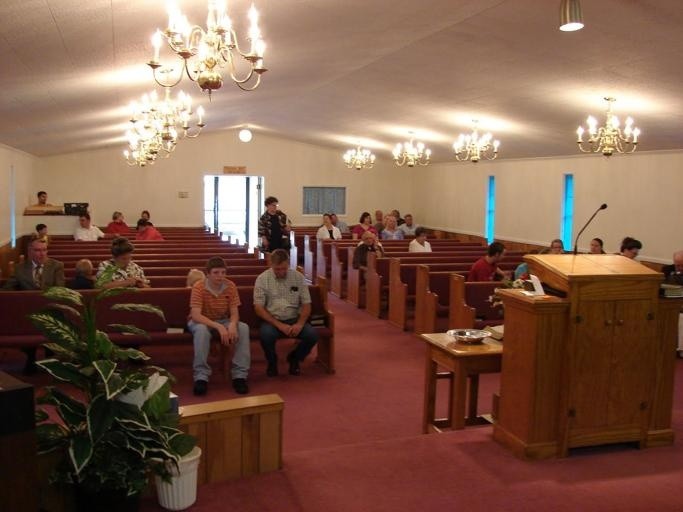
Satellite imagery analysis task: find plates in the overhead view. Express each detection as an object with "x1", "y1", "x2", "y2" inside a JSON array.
[{"x1": 447, "y1": 328, "x2": 492, "y2": 342}]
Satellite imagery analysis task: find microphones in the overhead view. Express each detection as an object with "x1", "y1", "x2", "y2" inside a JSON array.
[{"x1": 572, "y1": 201, "x2": 608, "y2": 253}]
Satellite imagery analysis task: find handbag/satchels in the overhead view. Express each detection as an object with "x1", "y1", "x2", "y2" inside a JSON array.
[{"x1": 279, "y1": 238, "x2": 288, "y2": 247}]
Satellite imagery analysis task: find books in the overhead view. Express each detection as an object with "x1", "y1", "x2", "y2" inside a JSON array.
[
  {"x1": 308, "y1": 313, "x2": 326, "y2": 325},
  {"x1": 661, "y1": 283, "x2": 683, "y2": 296}
]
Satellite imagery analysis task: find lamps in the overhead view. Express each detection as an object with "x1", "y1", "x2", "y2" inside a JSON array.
[
  {"x1": 392, "y1": 130, "x2": 432, "y2": 167},
  {"x1": 576, "y1": 97, "x2": 641, "y2": 158},
  {"x1": 128, "y1": 141, "x2": 177, "y2": 160},
  {"x1": 342, "y1": 141, "x2": 376, "y2": 171},
  {"x1": 238, "y1": 128, "x2": 252, "y2": 143},
  {"x1": 558, "y1": 0, "x2": 585, "y2": 32},
  {"x1": 129, "y1": 87, "x2": 208, "y2": 142},
  {"x1": 122, "y1": 151, "x2": 155, "y2": 168},
  {"x1": 453, "y1": 112, "x2": 501, "y2": 162},
  {"x1": 147, "y1": 1, "x2": 268, "y2": 103}
]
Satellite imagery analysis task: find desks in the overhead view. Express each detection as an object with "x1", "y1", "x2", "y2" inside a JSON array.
[{"x1": 417, "y1": 332, "x2": 504, "y2": 434}]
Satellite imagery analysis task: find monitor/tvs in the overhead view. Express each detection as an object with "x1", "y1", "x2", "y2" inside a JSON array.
[{"x1": 63, "y1": 202, "x2": 89, "y2": 215}]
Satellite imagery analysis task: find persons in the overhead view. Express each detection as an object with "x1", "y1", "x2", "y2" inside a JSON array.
[
  {"x1": 589, "y1": 238, "x2": 606, "y2": 254},
  {"x1": 662, "y1": 250, "x2": 682, "y2": 281},
  {"x1": 186, "y1": 255, "x2": 251, "y2": 396},
  {"x1": 515, "y1": 238, "x2": 565, "y2": 280},
  {"x1": 186, "y1": 268, "x2": 206, "y2": 288},
  {"x1": 464, "y1": 242, "x2": 512, "y2": 319},
  {"x1": 258, "y1": 196, "x2": 292, "y2": 253},
  {"x1": 620, "y1": 236, "x2": 643, "y2": 260},
  {"x1": 0, "y1": 190, "x2": 165, "y2": 378},
  {"x1": 253, "y1": 249, "x2": 319, "y2": 378},
  {"x1": 316, "y1": 209, "x2": 432, "y2": 283}
]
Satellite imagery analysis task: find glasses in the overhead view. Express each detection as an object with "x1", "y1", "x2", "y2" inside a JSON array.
[
  {"x1": 31, "y1": 248, "x2": 47, "y2": 253},
  {"x1": 627, "y1": 249, "x2": 638, "y2": 255}
]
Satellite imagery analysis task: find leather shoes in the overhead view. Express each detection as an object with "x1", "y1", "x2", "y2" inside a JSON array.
[
  {"x1": 266, "y1": 354, "x2": 278, "y2": 377},
  {"x1": 286, "y1": 352, "x2": 300, "y2": 375}
]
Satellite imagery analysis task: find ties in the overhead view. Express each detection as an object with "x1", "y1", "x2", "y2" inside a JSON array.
[
  {"x1": 34, "y1": 266, "x2": 41, "y2": 289},
  {"x1": 269, "y1": 203, "x2": 279, "y2": 207}
]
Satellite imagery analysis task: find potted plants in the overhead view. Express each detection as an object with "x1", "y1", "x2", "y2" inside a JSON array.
[{"x1": 33, "y1": 285, "x2": 202, "y2": 510}]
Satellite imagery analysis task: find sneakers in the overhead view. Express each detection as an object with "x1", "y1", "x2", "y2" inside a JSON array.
[
  {"x1": 193, "y1": 379, "x2": 207, "y2": 395},
  {"x1": 232, "y1": 377, "x2": 248, "y2": 394}
]
